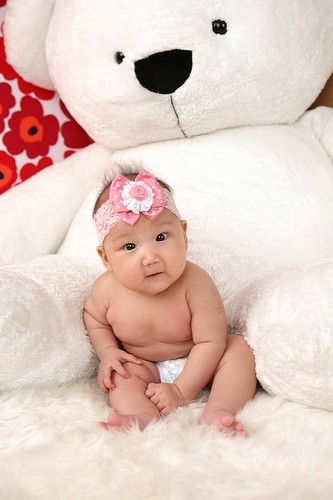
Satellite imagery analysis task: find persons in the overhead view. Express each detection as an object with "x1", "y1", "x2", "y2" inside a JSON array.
[{"x1": 82, "y1": 171, "x2": 257, "y2": 439}]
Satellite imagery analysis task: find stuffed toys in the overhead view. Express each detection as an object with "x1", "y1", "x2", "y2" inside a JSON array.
[{"x1": 0, "y1": 0, "x2": 333, "y2": 414}]
